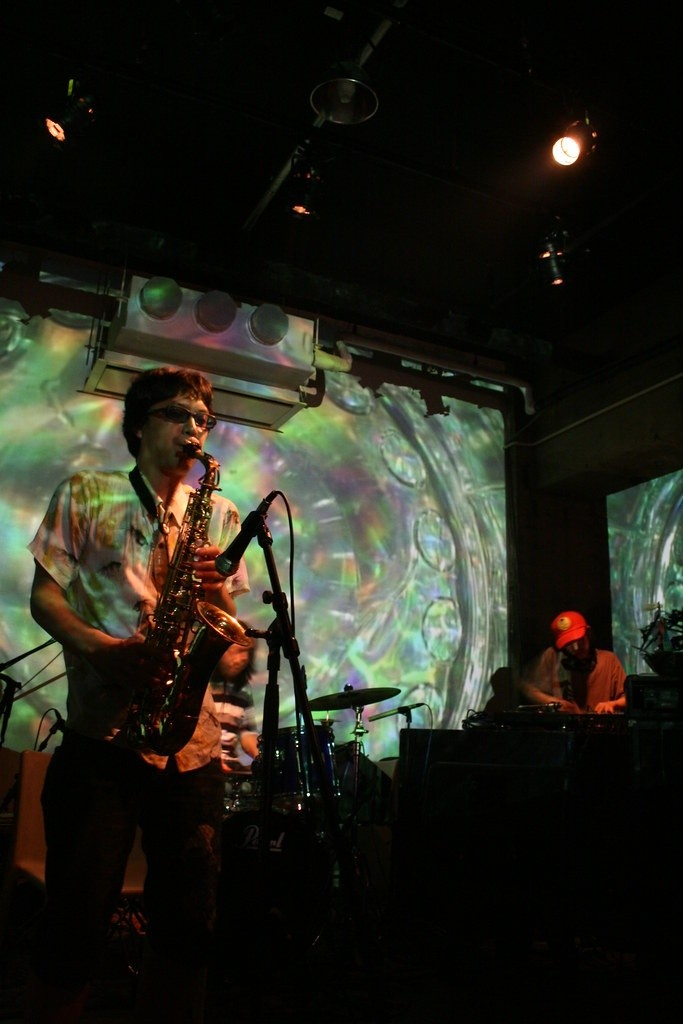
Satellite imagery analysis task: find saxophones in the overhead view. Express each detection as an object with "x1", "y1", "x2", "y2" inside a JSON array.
[{"x1": 115, "y1": 439, "x2": 256, "y2": 755}]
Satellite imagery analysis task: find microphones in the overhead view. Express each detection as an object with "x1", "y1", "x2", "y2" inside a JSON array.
[
  {"x1": 367, "y1": 703, "x2": 424, "y2": 722},
  {"x1": 54, "y1": 709, "x2": 65, "y2": 732},
  {"x1": 215, "y1": 489, "x2": 277, "y2": 577}
]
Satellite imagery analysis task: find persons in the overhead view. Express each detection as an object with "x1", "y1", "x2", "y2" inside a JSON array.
[
  {"x1": 522, "y1": 612, "x2": 626, "y2": 714},
  {"x1": 29, "y1": 367, "x2": 248, "y2": 1023},
  {"x1": 209, "y1": 618, "x2": 263, "y2": 802}
]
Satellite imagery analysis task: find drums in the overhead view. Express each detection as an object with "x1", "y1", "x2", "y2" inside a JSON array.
[
  {"x1": 258, "y1": 725, "x2": 342, "y2": 816},
  {"x1": 221, "y1": 771, "x2": 259, "y2": 813}
]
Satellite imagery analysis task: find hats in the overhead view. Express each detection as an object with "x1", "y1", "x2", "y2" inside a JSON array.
[{"x1": 551, "y1": 611, "x2": 590, "y2": 650}]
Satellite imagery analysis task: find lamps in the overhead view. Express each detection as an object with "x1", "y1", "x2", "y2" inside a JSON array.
[
  {"x1": 531, "y1": 217, "x2": 571, "y2": 286},
  {"x1": 310, "y1": 58, "x2": 380, "y2": 125},
  {"x1": 45, "y1": 70, "x2": 98, "y2": 143},
  {"x1": 289, "y1": 149, "x2": 327, "y2": 216}
]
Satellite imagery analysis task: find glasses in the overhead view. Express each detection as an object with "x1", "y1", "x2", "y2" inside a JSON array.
[{"x1": 147, "y1": 404, "x2": 217, "y2": 429}]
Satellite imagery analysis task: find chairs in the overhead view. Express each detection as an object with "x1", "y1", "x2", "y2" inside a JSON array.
[{"x1": 0, "y1": 751, "x2": 148, "y2": 975}]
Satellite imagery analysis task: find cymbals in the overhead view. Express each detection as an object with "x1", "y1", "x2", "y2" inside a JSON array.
[
  {"x1": 296, "y1": 687, "x2": 401, "y2": 711},
  {"x1": 313, "y1": 718, "x2": 341, "y2": 722}
]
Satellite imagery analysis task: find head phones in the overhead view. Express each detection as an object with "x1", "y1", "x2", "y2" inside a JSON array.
[{"x1": 562, "y1": 647, "x2": 596, "y2": 676}]
